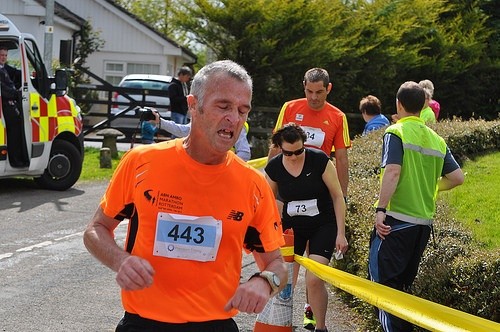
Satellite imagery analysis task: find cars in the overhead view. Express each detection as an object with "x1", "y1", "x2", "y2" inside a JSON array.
[{"x1": 111, "y1": 74, "x2": 191, "y2": 138}]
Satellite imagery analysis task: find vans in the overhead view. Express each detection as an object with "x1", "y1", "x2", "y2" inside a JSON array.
[{"x1": 0, "y1": 12, "x2": 85, "y2": 191}]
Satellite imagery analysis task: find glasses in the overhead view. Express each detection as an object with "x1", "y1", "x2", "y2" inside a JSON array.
[{"x1": 281, "y1": 146, "x2": 304, "y2": 156}]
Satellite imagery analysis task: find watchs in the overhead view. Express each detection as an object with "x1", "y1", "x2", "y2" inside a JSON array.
[
  {"x1": 344, "y1": 196, "x2": 348, "y2": 203},
  {"x1": 249, "y1": 270, "x2": 281, "y2": 296},
  {"x1": 374, "y1": 206, "x2": 386, "y2": 213}
]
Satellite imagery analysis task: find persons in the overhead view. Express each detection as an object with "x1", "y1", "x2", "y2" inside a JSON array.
[
  {"x1": 0, "y1": 48, "x2": 29, "y2": 168},
  {"x1": 418, "y1": 79, "x2": 440, "y2": 120},
  {"x1": 149, "y1": 109, "x2": 252, "y2": 161},
  {"x1": 263, "y1": 124, "x2": 349, "y2": 332},
  {"x1": 167, "y1": 66, "x2": 193, "y2": 139},
  {"x1": 391, "y1": 86, "x2": 436, "y2": 124},
  {"x1": 265, "y1": 67, "x2": 352, "y2": 211},
  {"x1": 367, "y1": 81, "x2": 465, "y2": 332},
  {"x1": 359, "y1": 94, "x2": 391, "y2": 137},
  {"x1": 82, "y1": 59, "x2": 290, "y2": 331}
]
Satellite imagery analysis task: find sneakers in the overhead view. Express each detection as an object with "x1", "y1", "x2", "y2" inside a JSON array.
[{"x1": 303, "y1": 304, "x2": 316, "y2": 330}]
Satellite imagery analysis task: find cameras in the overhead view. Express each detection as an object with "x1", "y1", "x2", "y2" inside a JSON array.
[{"x1": 134, "y1": 106, "x2": 159, "y2": 121}]
